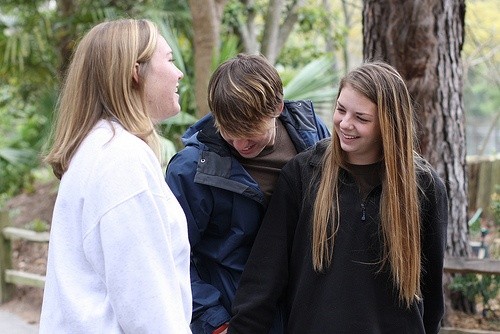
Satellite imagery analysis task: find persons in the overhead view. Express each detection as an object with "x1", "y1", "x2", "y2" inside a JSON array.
[
  {"x1": 37, "y1": 17, "x2": 193, "y2": 333},
  {"x1": 165, "y1": 53, "x2": 331, "y2": 333},
  {"x1": 226, "y1": 58, "x2": 448, "y2": 334}
]
